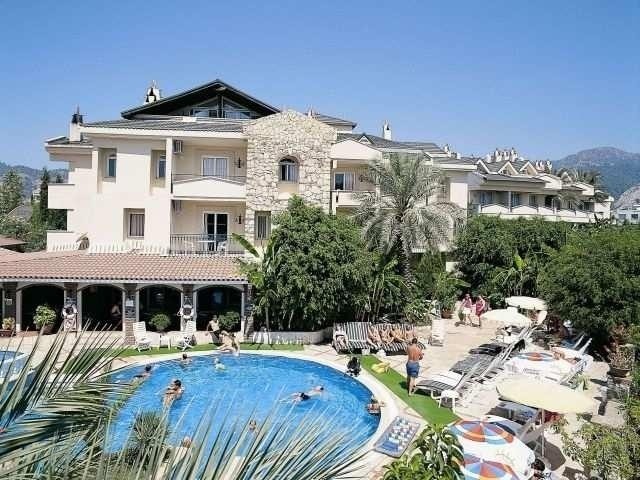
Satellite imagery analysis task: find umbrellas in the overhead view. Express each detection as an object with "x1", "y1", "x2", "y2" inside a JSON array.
[
  {"x1": 479, "y1": 308, "x2": 532, "y2": 352},
  {"x1": 497, "y1": 374, "x2": 602, "y2": 458},
  {"x1": 449, "y1": 417, "x2": 514, "y2": 446},
  {"x1": 505, "y1": 296, "x2": 549, "y2": 317},
  {"x1": 461, "y1": 454, "x2": 516, "y2": 479}
]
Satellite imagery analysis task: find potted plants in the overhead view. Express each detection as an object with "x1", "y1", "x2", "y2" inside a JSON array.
[
  {"x1": 608, "y1": 340, "x2": 636, "y2": 379},
  {"x1": 33, "y1": 305, "x2": 56, "y2": 335},
  {"x1": 0, "y1": 317, "x2": 17, "y2": 337}
]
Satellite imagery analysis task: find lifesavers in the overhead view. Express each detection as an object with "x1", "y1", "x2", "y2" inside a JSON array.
[
  {"x1": 372, "y1": 362, "x2": 390, "y2": 374},
  {"x1": 62, "y1": 304, "x2": 77, "y2": 319},
  {"x1": 136, "y1": 336, "x2": 152, "y2": 344},
  {"x1": 180, "y1": 304, "x2": 194, "y2": 319}
]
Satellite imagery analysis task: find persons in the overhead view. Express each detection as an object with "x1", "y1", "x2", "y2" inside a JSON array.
[
  {"x1": 332, "y1": 322, "x2": 425, "y2": 397},
  {"x1": 204, "y1": 315, "x2": 240, "y2": 357},
  {"x1": 157, "y1": 379, "x2": 183, "y2": 409},
  {"x1": 110, "y1": 303, "x2": 122, "y2": 318},
  {"x1": 181, "y1": 354, "x2": 192, "y2": 366},
  {"x1": 278, "y1": 386, "x2": 324, "y2": 405},
  {"x1": 471, "y1": 293, "x2": 485, "y2": 326},
  {"x1": 249, "y1": 419, "x2": 257, "y2": 431},
  {"x1": 367, "y1": 397, "x2": 385, "y2": 415},
  {"x1": 144, "y1": 365, "x2": 153, "y2": 378},
  {"x1": 215, "y1": 360, "x2": 226, "y2": 371},
  {"x1": 459, "y1": 293, "x2": 473, "y2": 326}
]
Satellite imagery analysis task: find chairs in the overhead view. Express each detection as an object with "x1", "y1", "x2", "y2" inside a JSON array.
[
  {"x1": 332, "y1": 312, "x2": 547, "y2": 445},
  {"x1": 131, "y1": 319, "x2": 153, "y2": 353},
  {"x1": 547, "y1": 335, "x2": 593, "y2": 387},
  {"x1": 177, "y1": 320, "x2": 196, "y2": 352},
  {"x1": 182, "y1": 238, "x2": 231, "y2": 256}
]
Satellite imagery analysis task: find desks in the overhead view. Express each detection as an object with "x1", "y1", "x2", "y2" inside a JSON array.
[{"x1": 157, "y1": 334, "x2": 171, "y2": 351}]
